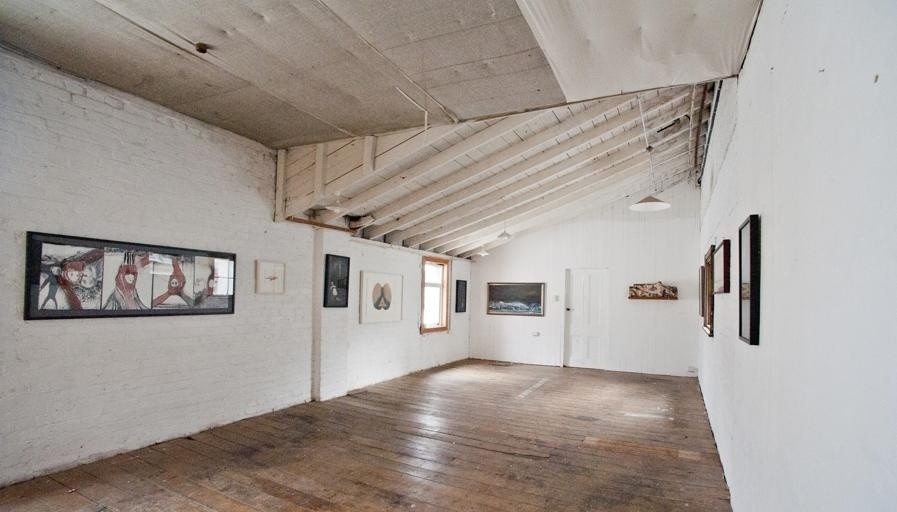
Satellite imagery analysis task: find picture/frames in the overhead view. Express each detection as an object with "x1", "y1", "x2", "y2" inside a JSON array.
[
  {"x1": 356, "y1": 269, "x2": 406, "y2": 324},
  {"x1": 252, "y1": 258, "x2": 285, "y2": 295},
  {"x1": 455, "y1": 279, "x2": 466, "y2": 313},
  {"x1": 483, "y1": 280, "x2": 546, "y2": 317},
  {"x1": 322, "y1": 252, "x2": 351, "y2": 310},
  {"x1": 694, "y1": 213, "x2": 761, "y2": 347},
  {"x1": 19, "y1": 226, "x2": 237, "y2": 322}
]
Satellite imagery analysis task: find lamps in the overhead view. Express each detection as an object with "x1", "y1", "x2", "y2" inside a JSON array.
[{"x1": 629, "y1": 138, "x2": 674, "y2": 217}]
